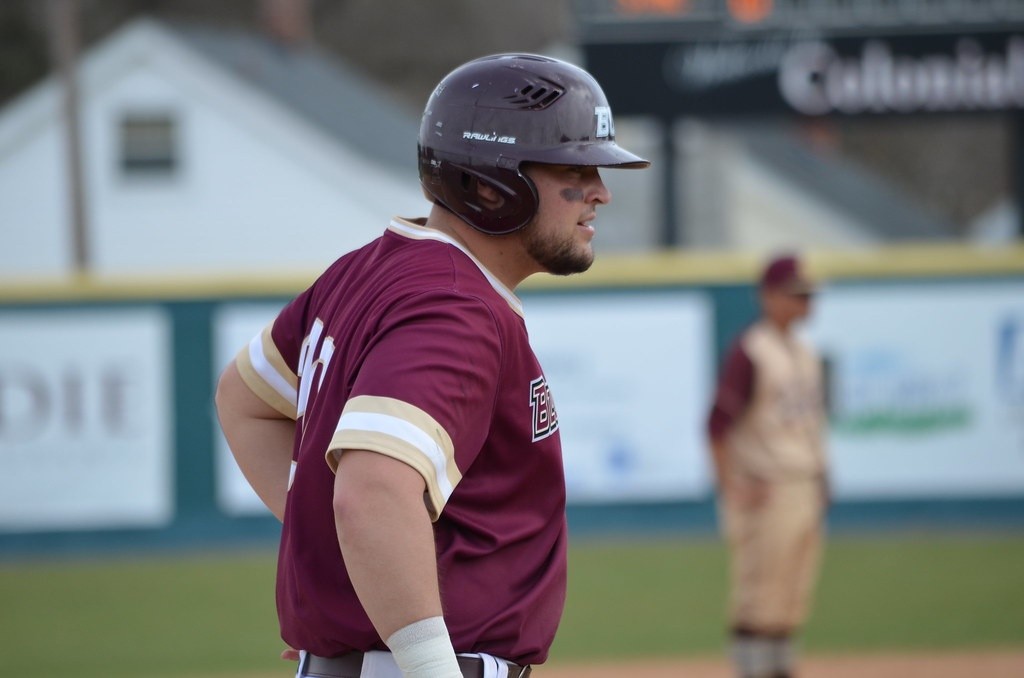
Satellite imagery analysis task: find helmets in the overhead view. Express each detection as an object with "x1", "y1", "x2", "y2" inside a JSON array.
[{"x1": 418, "y1": 52, "x2": 650, "y2": 236}]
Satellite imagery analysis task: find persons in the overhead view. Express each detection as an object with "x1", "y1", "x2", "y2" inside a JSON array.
[
  {"x1": 215, "y1": 53, "x2": 650, "y2": 678},
  {"x1": 707, "y1": 257, "x2": 827, "y2": 678}
]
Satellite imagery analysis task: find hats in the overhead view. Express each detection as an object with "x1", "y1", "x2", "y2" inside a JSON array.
[{"x1": 762, "y1": 258, "x2": 821, "y2": 295}]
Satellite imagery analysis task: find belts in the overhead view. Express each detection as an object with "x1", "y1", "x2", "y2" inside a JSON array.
[{"x1": 296, "y1": 655, "x2": 532, "y2": 678}]
